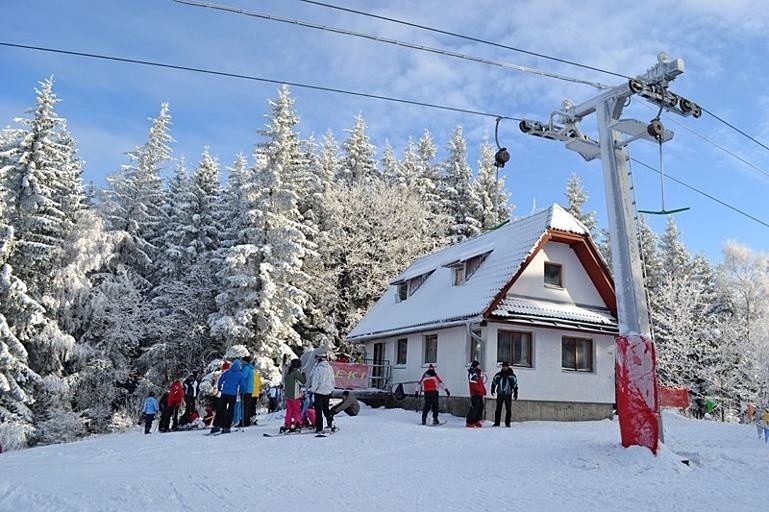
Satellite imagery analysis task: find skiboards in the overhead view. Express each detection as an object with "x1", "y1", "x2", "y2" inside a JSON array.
[
  {"x1": 419, "y1": 423, "x2": 445, "y2": 426},
  {"x1": 202, "y1": 429, "x2": 238, "y2": 436}
]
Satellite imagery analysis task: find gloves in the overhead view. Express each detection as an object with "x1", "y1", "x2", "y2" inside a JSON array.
[
  {"x1": 491, "y1": 391, "x2": 495, "y2": 396},
  {"x1": 445, "y1": 389, "x2": 450, "y2": 397},
  {"x1": 415, "y1": 392, "x2": 418, "y2": 398},
  {"x1": 513, "y1": 394, "x2": 517, "y2": 401}
]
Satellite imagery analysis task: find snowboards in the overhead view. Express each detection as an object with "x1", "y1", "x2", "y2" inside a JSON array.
[
  {"x1": 263, "y1": 430, "x2": 315, "y2": 437},
  {"x1": 315, "y1": 427, "x2": 339, "y2": 437}
]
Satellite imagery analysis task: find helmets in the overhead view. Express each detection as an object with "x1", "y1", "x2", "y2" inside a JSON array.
[{"x1": 315, "y1": 353, "x2": 327, "y2": 361}]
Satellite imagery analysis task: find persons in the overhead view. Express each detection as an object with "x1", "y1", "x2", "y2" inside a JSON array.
[
  {"x1": 140, "y1": 351, "x2": 360, "y2": 435},
  {"x1": 756, "y1": 404, "x2": 762, "y2": 439},
  {"x1": 463, "y1": 361, "x2": 487, "y2": 429},
  {"x1": 415, "y1": 364, "x2": 451, "y2": 427},
  {"x1": 744, "y1": 404, "x2": 753, "y2": 422},
  {"x1": 490, "y1": 361, "x2": 519, "y2": 427},
  {"x1": 762, "y1": 407, "x2": 769, "y2": 443}
]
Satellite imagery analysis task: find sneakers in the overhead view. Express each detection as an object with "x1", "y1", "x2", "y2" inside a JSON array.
[
  {"x1": 433, "y1": 420, "x2": 439, "y2": 424},
  {"x1": 506, "y1": 424, "x2": 510, "y2": 427},
  {"x1": 422, "y1": 419, "x2": 426, "y2": 424},
  {"x1": 466, "y1": 423, "x2": 474, "y2": 427},
  {"x1": 492, "y1": 424, "x2": 500, "y2": 427},
  {"x1": 474, "y1": 421, "x2": 481, "y2": 427}
]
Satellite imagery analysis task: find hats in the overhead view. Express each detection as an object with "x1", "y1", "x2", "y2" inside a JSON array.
[
  {"x1": 500, "y1": 362, "x2": 511, "y2": 366},
  {"x1": 429, "y1": 364, "x2": 434, "y2": 371},
  {"x1": 291, "y1": 359, "x2": 301, "y2": 368}
]
